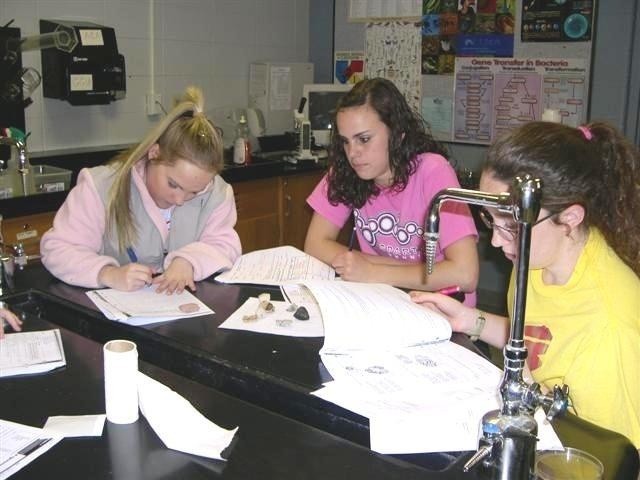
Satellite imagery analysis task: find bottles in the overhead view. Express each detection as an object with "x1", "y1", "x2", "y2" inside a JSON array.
[
  {"x1": 0, "y1": 159, "x2": 22, "y2": 199},
  {"x1": 1, "y1": 66, "x2": 41, "y2": 105}
]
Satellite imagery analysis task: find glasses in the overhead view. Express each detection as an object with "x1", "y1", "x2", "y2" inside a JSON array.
[{"x1": 479, "y1": 209, "x2": 553, "y2": 242}]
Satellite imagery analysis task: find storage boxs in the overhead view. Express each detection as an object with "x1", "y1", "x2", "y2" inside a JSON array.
[{"x1": 32, "y1": 165, "x2": 73, "y2": 194}]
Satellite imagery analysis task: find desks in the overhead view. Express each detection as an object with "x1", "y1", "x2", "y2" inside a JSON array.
[
  {"x1": 0, "y1": 257, "x2": 639, "y2": 480},
  {"x1": 0, "y1": 308, "x2": 449, "y2": 480}
]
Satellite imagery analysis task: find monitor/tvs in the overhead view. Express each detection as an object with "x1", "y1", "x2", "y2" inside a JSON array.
[{"x1": 302, "y1": 83, "x2": 354, "y2": 159}]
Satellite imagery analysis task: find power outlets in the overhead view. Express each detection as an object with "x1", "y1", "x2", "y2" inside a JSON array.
[{"x1": 146, "y1": 94, "x2": 163, "y2": 116}]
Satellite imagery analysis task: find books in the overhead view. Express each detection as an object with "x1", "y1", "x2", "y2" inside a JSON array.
[{"x1": 301, "y1": 283, "x2": 565, "y2": 453}]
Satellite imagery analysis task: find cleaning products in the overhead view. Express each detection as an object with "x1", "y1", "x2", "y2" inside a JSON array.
[{"x1": 1, "y1": 127, "x2": 37, "y2": 198}]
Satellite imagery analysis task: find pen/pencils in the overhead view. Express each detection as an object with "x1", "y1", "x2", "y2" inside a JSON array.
[
  {"x1": 127, "y1": 248, "x2": 152, "y2": 288},
  {"x1": 349, "y1": 227, "x2": 357, "y2": 251},
  {"x1": 438, "y1": 286, "x2": 460, "y2": 295}
]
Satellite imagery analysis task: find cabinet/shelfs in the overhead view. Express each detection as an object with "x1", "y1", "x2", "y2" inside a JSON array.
[
  {"x1": 229, "y1": 173, "x2": 326, "y2": 253},
  {"x1": 0, "y1": 209, "x2": 57, "y2": 263}
]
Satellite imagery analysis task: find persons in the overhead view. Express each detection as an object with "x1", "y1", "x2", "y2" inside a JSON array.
[
  {"x1": 0, "y1": 307, "x2": 24, "y2": 338},
  {"x1": 409, "y1": 121, "x2": 640, "y2": 454},
  {"x1": 40, "y1": 88, "x2": 242, "y2": 295},
  {"x1": 303, "y1": 77, "x2": 477, "y2": 306}
]
